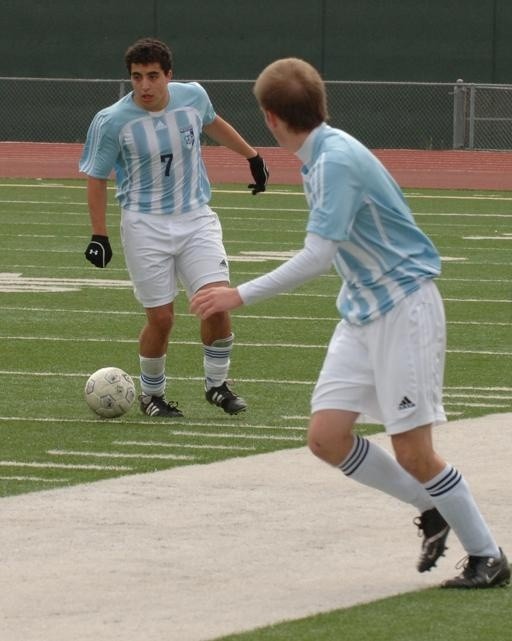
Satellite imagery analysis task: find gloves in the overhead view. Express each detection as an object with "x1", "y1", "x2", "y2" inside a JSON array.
[
  {"x1": 247, "y1": 153, "x2": 269, "y2": 196},
  {"x1": 84, "y1": 233, "x2": 113, "y2": 266}
]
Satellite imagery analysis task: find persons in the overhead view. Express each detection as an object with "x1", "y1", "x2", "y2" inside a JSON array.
[
  {"x1": 77, "y1": 39, "x2": 269, "y2": 415},
  {"x1": 189, "y1": 56, "x2": 511, "y2": 587}
]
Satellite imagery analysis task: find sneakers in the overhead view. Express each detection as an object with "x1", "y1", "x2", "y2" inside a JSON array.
[
  {"x1": 440, "y1": 547, "x2": 511, "y2": 590},
  {"x1": 413, "y1": 508, "x2": 450, "y2": 573},
  {"x1": 205, "y1": 382, "x2": 248, "y2": 415},
  {"x1": 138, "y1": 394, "x2": 182, "y2": 417}
]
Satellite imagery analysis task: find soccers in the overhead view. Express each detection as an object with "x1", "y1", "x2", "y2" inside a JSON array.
[{"x1": 84, "y1": 366, "x2": 135, "y2": 417}]
